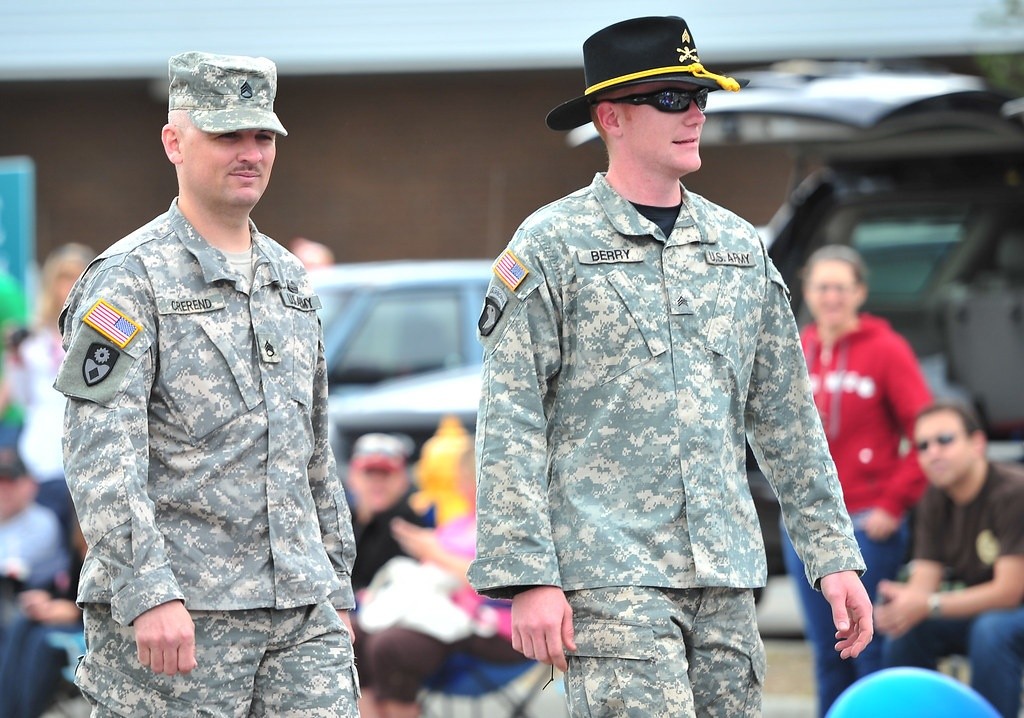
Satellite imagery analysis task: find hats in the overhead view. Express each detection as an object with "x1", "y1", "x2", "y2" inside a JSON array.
[
  {"x1": 168, "y1": 52, "x2": 289, "y2": 136},
  {"x1": 354, "y1": 433, "x2": 405, "y2": 471},
  {"x1": 545, "y1": 16, "x2": 751, "y2": 131},
  {"x1": 0, "y1": 447, "x2": 24, "y2": 481}
]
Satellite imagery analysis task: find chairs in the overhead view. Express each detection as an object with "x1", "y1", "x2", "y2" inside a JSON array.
[{"x1": 415, "y1": 653, "x2": 559, "y2": 718}]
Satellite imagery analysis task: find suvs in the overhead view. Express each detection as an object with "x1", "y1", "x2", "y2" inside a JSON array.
[{"x1": 571, "y1": 67, "x2": 1023, "y2": 576}]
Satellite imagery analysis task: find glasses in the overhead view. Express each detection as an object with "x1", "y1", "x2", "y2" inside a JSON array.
[
  {"x1": 592, "y1": 88, "x2": 708, "y2": 113},
  {"x1": 916, "y1": 432, "x2": 955, "y2": 451}
]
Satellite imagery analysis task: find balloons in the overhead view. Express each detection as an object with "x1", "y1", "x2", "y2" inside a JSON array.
[{"x1": 825, "y1": 667, "x2": 1004, "y2": 718}]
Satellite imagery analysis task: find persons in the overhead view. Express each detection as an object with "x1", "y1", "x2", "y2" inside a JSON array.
[
  {"x1": 778, "y1": 244, "x2": 938, "y2": 718},
  {"x1": 465, "y1": 13, "x2": 876, "y2": 718},
  {"x1": 0, "y1": 236, "x2": 568, "y2": 718},
  {"x1": 52, "y1": 50, "x2": 364, "y2": 718},
  {"x1": 873, "y1": 405, "x2": 1024, "y2": 718}
]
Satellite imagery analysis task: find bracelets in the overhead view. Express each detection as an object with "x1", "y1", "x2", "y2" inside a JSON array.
[{"x1": 930, "y1": 590, "x2": 941, "y2": 620}]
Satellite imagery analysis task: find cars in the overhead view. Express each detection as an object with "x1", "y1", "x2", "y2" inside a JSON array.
[{"x1": 21, "y1": 259, "x2": 495, "y2": 513}]
